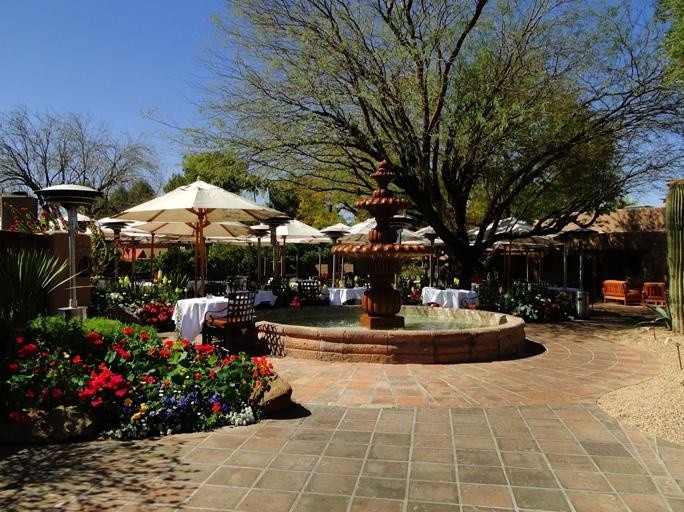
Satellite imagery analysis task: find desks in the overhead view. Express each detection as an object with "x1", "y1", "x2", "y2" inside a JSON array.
[
  {"x1": 422, "y1": 287, "x2": 479, "y2": 308},
  {"x1": 234, "y1": 291, "x2": 278, "y2": 312},
  {"x1": 329, "y1": 287, "x2": 367, "y2": 305},
  {"x1": 173, "y1": 296, "x2": 254, "y2": 345}
]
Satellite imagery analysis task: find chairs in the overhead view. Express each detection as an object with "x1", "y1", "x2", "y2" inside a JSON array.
[
  {"x1": 461, "y1": 289, "x2": 500, "y2": 311},
  {"x1": 640, "y1": 282, "x2": 666, "y2": 308},
  {"x1": 205, "y1": 292, "x2": 259, "y2": 349},
  {"x1": 295, "y1": 280, "x2": 324, "y2": 306}
]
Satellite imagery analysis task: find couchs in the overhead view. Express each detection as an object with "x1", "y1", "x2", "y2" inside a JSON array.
[{"x1": 601, "y1": 279, "x2": 641, "y2": 305}]
